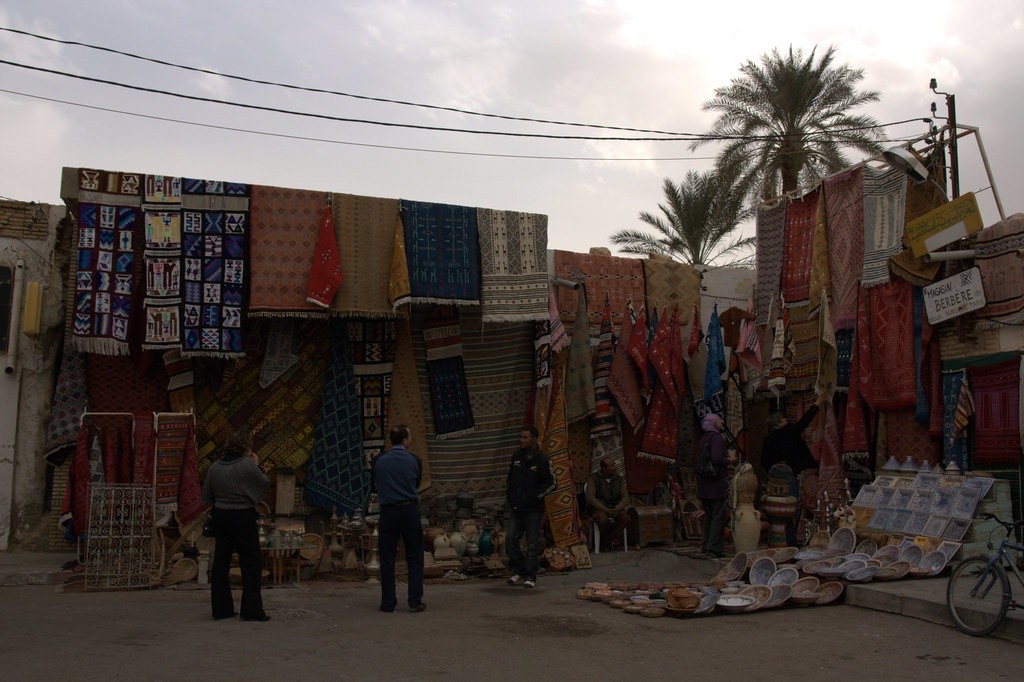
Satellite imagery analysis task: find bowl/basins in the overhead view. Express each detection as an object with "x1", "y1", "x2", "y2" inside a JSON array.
[{"x1": 578, "y1": 523, "x2": 945, "y2": 618}]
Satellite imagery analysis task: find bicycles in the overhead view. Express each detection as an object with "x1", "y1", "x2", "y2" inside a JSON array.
[{"x1": 946, "y1": 512, "x2": 1024, "y2": 637}]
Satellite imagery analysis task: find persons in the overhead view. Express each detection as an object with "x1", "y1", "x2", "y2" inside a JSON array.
[
  {"x1": 373, "y1": 425, "x2": 428, "y2": 613},
  {"x1": 505, "y1": 427, "x2": 557, "y2": 588},
  {"x1": 760, "y1": 398, "x2": 822, "y2": 548},
  {"x1": 585, "y1": 458, "x2": 632, "y2": 552},
  {"x1": 696, "y1": 413, "x2": 736, "y2": 559},
  {"x1": 202, "y1": 430, "x2": 273, "y2": 621}
]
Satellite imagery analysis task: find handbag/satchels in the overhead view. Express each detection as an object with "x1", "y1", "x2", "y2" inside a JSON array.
[
  {"x1": 515, "y1": 484, "x2": 545, "y2": 512},
  {"x1": 694, "y1": 434, "x2": 721, "y2": 481},
  {"x1": 202, "y1": 517, "x2": 215, "y2": 538}
]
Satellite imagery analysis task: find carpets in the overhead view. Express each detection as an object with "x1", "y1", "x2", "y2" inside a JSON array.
[{"x1": 54, "y1": 146, "x2": 1024, "y2": 549}]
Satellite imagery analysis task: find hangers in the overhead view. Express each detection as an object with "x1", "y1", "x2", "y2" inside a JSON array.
[{"x1": 729, "y1": 298, "x2": 738, "y2": 311}]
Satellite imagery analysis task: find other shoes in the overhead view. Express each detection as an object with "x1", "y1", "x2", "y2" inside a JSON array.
[
  {"x1": 379, "y1": 604, "x2": 395, "y2": 612},
  {"x1": 700, "y1": 547, "x2": 728, "y2": 560},
  {"x1": 508, "y1": 575, "x2": 521, "y2": 585},
  {"x1": 524, "y1": 579, "x2": 535, "y2": 589},
  {"x1": 215, "y1": 613, "x2": 238, "y2": 619},
  {"x1": 408, "y1": 602, "x2": 429, "y2": 613},
  {"x1": 245, "y1": 613, "x2": 271, "y2": 622}
]
